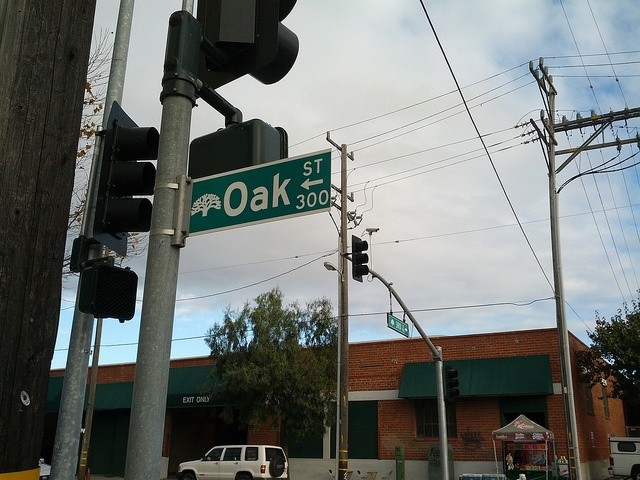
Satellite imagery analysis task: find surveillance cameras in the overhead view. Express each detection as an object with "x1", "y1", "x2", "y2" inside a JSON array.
[{"x1": 366, "y1": 227, "x2": 380, "y2": 232}]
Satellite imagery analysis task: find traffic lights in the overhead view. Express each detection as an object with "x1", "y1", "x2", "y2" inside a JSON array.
[
  {"x1": 351, "y1": 235, "x2": 369, "y2": 283},
  {"x1": 445, "y1": 368, "x2": 460, "y2": 399},
  {"x1": 93, "y1": 101, "x2": 160, "y2": 258}
]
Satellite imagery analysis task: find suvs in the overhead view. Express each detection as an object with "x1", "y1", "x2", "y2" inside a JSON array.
[{"x1": 177, "y1": 444, "x2": 289, "y2": 480}]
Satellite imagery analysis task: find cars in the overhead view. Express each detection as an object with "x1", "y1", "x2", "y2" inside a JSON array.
[{"x1": 609, "y1": 436, "x2": 640, "y2": 480}]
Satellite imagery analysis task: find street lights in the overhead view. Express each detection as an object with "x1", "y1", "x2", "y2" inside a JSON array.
[{"x1": 323, "y1": 262, "x2": 349, "y2": 480}]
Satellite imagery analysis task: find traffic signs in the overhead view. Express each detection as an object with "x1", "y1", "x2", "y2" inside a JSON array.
[
  {"x1": 189, "y1": 148, "x2": 332, "y2": 237},
  {"x1": 387, "y1": 312, "x2": 409, "y2": 337}
]
